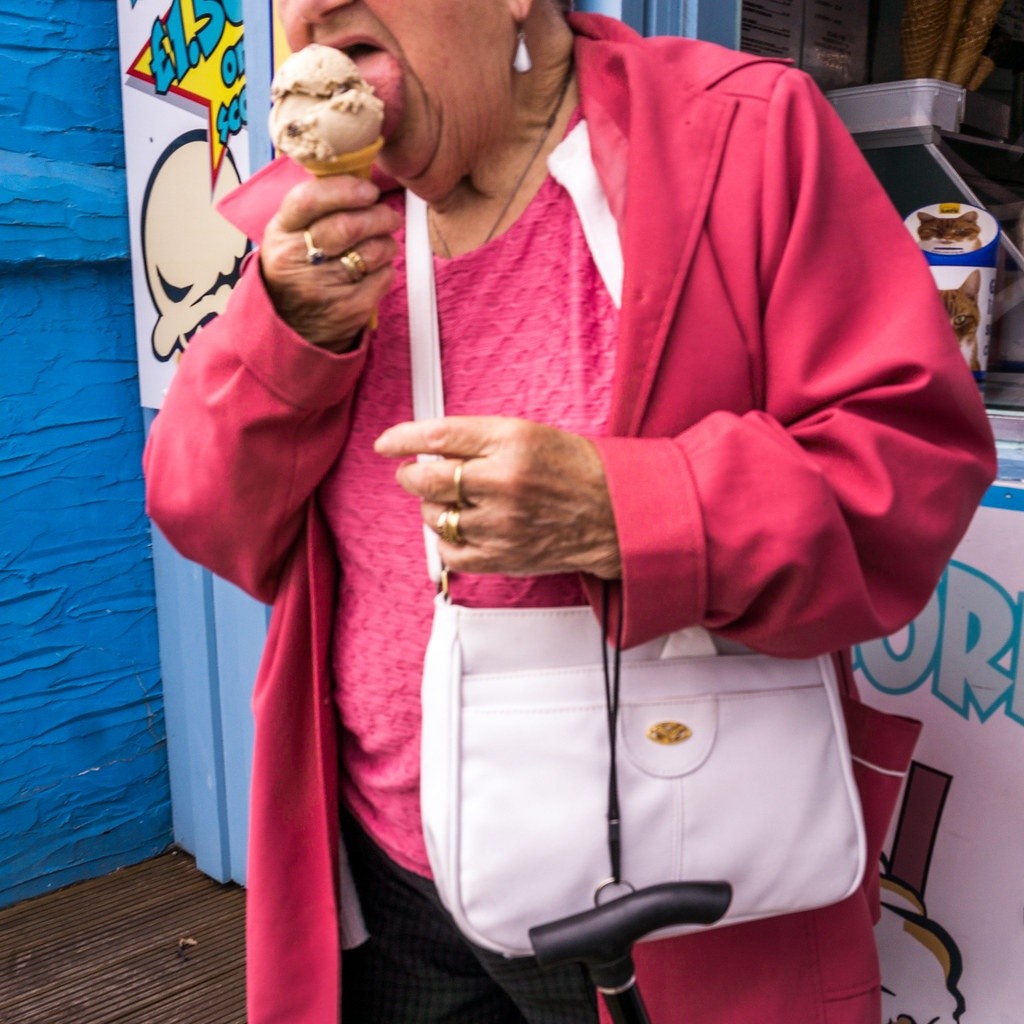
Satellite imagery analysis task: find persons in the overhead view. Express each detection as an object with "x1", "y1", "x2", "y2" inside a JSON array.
[{"x1": 142, "y1": 0, "x2": 998, "y2": 1024}]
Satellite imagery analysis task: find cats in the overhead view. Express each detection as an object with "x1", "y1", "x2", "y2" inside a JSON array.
[
  {"x1": 917, "y1": 211, "x2": 982, "y2": 254},
  {"x1": 935, "y1": 269, "x2": 982, "y2": 370}
]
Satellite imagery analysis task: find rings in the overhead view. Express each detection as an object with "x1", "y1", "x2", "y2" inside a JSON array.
[
  {"x1": 303, "y1": 231, "x2": 325, "y2": 265},
  {"x1": 437, "y1": 509, "x2": 462, "y2": 543},
  {"x1": 454, "y1": 464, "x2": 467, "y2": 507},
  {"x1": 339, "y1": 250, "x2": 365, "y2": 280}
]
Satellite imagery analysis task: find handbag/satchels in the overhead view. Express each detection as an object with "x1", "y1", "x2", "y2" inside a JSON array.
[{"x1": 420, "y1": 592, "x2": 869, "y2": 957}]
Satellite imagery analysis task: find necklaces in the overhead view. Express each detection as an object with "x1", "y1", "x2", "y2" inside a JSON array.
[{"x1": 430, "y1": 53, "x2": 574, "y2": 259}]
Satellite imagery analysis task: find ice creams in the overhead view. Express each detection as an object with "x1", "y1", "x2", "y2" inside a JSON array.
[{"x1": 267, "y1": 42, "x2": 386, "y2": 329}]
